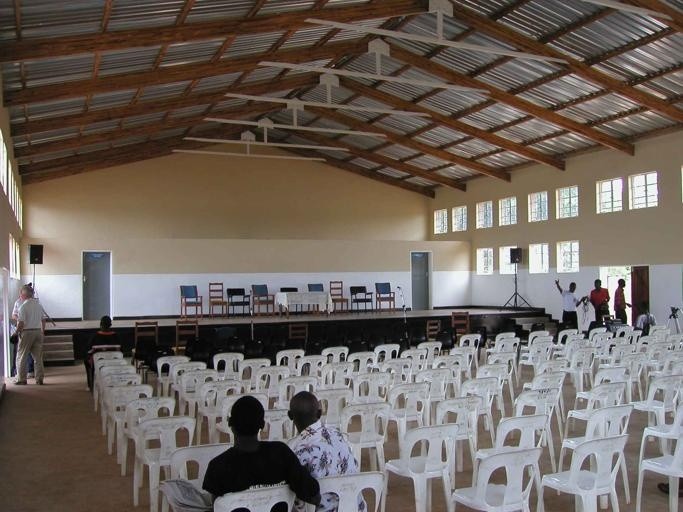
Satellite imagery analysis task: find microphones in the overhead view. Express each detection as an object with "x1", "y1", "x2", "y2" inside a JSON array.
[{"x1": 397, "y1": 286, "x2": 403, "y2": 292}]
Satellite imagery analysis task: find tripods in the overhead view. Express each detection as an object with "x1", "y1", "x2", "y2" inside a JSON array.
[
  {"x1": 33, "y1": 264, "x2": 56, "y2": 326},
  {"x1": 500, "y1": 263, "x2": 535, "y2": 312}
]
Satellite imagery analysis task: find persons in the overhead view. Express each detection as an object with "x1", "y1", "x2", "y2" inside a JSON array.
[
  {"x1": 198, "y1": 395, "x2": 323, "y2": 511},
  {"x1": 612, "y1": 278, "x2": 628, "y2": 327},
  {"x1": 629, "y1": 302, "x2": 656, "y2": 343},
  {"x1": 84, "y1": 315, "x2": 121, "y2": 392},
  {"x1": 11, "y1": 282, "x2": 35, "y2": 379},
  {"x1": 584, "y1": 279, "x2": 610, "y2": 327},
  {"x1": 657, "y1": 476, "x2": 682, "y2": 499},
  {"x1": 13, "y1": 286, "x2": 47, "y2": 385},
  {"x1": 284, "y1": 389, "x2": 370, "y2": 512},
  {"x1": 554, "y1": 278, "x2": 588, "y2": 331}
]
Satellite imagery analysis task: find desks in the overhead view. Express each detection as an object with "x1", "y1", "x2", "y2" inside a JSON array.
[{"x1": 277, "y1": 292, "x2": 329, "y2": 316}]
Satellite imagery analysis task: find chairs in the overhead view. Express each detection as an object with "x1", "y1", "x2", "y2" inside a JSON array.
[
  {"x1": 180, "y1": 285, "x2": 202, "y2": 320},
  {"x1": 308, "y1": 284, "x2": 327, "y2": 314},
  {"x1": 329, "y1": 282, "x2": 348, "y2": 316},
  {"x1": 350, "y1": 286, "x2": 373, "y2": 311},
  {"x1": 282, "y1": 288, "x2": 302, "y2": 315},
  {"x1": 91, "y1": 326, "x2": 683, "y2": 511},
  {"x1": 208, "y1": 283, "x2": 228, "y2": 320},
  {"x1": 226, "y1": 288, "x2": 251, "y2": 317},
  {"x1": 251, "y1": 284, "x2": 276, "y2": 318},
  {"x1": 375, "y1": 283, "x2": 396, "y2": 313}
]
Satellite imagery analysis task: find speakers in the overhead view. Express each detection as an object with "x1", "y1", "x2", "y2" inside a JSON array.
[
  {"x1": 30, "y1": 245, "x2": 43, "y2": 264},
  {"x1": 510, "y1": 248, "x2": 522, "y2": 263}
]
